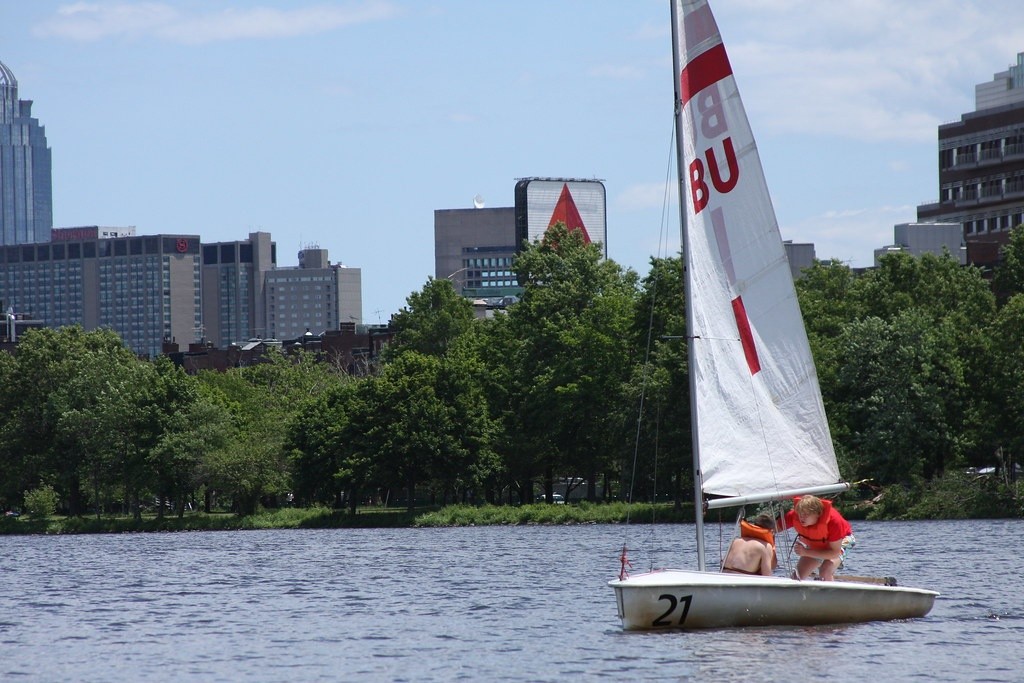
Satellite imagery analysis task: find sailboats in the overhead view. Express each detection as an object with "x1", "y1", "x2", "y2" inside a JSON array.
[{"x1": 606, "y1": 0, "x2": 942, "y2": 635}]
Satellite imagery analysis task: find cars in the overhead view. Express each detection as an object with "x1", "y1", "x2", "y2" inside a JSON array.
[{"x1": 536, "y1": 494, "x2": 569, "y2": 505}]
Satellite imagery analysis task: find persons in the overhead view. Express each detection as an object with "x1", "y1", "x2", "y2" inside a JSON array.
[
  {"x1": 286, "y1": 491, "x2": 294, "y2": 509},
  {"x1": 771, "y1": 495, "x2": 857, "y2": 582},
  {"x1": 722, "y1": 514, "x2": 775, "y2": 575}
]
